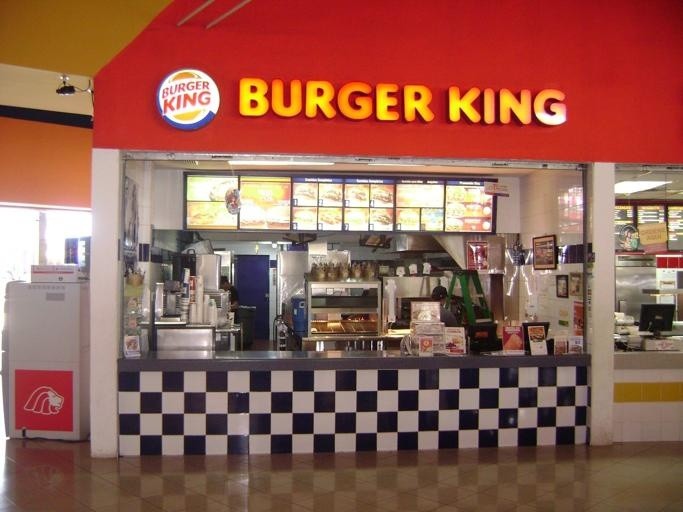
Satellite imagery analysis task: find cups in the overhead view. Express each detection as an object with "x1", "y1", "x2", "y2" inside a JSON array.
[
  {"x1": 180, "y1": 268, "x2": 203, "y2": 324},
  {"x1": 204, "y1": 294, "x2": 218, "y2": 324}
]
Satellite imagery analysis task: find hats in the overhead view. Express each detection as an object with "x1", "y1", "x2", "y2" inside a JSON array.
[{"x1": 433, "y1": 286, "x2": 449, "y2": 298}]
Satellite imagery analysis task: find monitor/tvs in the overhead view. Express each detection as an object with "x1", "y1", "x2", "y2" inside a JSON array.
[
  {"x1": 639, "y1": 304, "x2": 675, "y2": 340},
  {"x1": 522, "y1": 322, "x2": 550, "y2": 354}
]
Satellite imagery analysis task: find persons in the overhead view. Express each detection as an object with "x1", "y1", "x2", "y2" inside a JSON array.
[
  {"x1": 431, "y1": 286, "x2": 457, "y2": 327},
  {"x1": 220, "y1": 276, "x2": 239, "y2": 323}
]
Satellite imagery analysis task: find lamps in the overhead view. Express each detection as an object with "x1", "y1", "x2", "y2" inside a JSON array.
[{"x1": 466, "y1": 240, "x2": 489, "y2": 272}]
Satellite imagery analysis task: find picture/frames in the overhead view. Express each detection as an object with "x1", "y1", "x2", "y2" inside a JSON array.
[
  {"x1": 556, "y1": 274, "x2": 570, "y2": 299},
  {"x1": 532, "y1": 234, "x2": 559, "y2": 272}
]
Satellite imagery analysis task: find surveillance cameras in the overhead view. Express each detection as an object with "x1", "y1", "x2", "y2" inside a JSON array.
[{"x1": 56, "y1": 86, "x2": 75, "y2": 94}]
